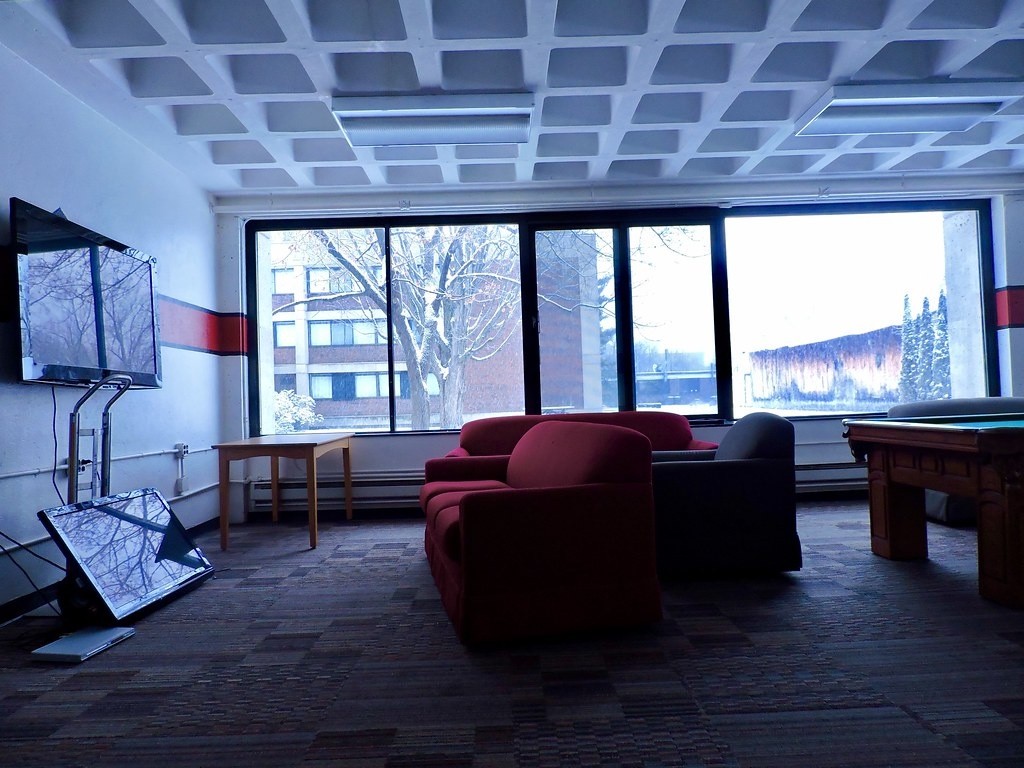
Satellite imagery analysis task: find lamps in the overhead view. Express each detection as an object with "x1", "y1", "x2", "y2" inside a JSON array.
[
  {"x1": 791, "y1": 81, "x2": 1024, "y2": 138},
  {"x1": 329, "y1": 91, "x2": 537, "y2": 148}
]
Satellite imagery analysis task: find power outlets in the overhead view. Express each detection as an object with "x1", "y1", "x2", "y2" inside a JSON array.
[
  {"x1": 66, "y1": 457, "x2": 86, "y2": 476},
  {"x1": 175, "y1": 442, "x2": 189, "y2": 458}
]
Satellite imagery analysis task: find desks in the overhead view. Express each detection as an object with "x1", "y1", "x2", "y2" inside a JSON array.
[
  {"x1": 842, "y1": 411, "x2": 1024, "y2": 610},
  {"x1": 211, "y1": 432, "x2": 357, "y2": 550}
]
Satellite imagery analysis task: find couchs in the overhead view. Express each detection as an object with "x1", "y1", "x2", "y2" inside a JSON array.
[
  {"x1": 442, "y1": 411, "x2": 719, "y2": 460},
  {"x1": 650, "y1": 413, "x2": 804, "y2": 573},
  {"x1": 417, "y1": 418, "x2": 654, "y2": 645},
  {"x1": 885, "y1": 395, "x2": 1024, "y2": 522}
]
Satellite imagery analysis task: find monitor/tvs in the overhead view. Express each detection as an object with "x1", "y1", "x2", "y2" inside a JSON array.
[
  {"x1": 9, "y1": 197, "x2": 163, "y2": 390},
  {"x1": 37, "y1": 487, "x2": 215, "y2": 621}
]
